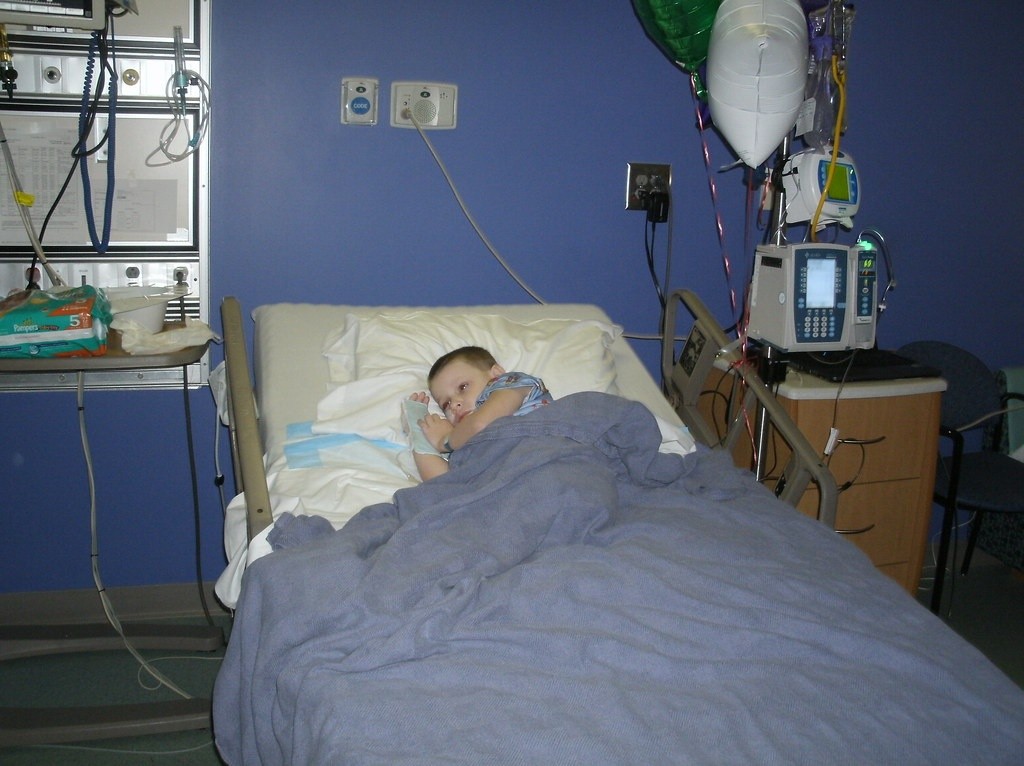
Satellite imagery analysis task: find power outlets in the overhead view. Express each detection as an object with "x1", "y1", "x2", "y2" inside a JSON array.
[
  {"x1": 126, "y1": 267, "x2": 140, "y2": 278},
  {"x1": 624, "y1": 163, "x2": 672, "y2": 212},
  {"x1": 173, "y1": 268, "x2": 188, "y2": 282},
  {"x1": 23, "y1": 264, "x2": 44, "y2": 290}
]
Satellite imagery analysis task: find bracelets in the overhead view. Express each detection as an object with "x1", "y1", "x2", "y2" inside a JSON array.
[{"x1": 444, "y1": 436, "x2": 454, "y2": 452}]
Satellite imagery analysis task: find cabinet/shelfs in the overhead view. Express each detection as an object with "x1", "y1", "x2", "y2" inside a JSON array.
[{"x1": 695, "y1": 346, "x2": 947, "y2": 601}]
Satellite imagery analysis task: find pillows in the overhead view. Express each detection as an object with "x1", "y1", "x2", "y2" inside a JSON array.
[{"x1": 311, "y1": 309, "x2": 625, "y2": 461}]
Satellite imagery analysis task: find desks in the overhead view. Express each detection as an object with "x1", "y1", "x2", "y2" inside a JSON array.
[{"x1": 0, "y1": 320, "x2": 209, "y2": 372}]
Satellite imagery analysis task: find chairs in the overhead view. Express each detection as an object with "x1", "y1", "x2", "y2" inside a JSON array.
[{"x1": 897, "y1": 341, "x2": 1024, "y2": 617}]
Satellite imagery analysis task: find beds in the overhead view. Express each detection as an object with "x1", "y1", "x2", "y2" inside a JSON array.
[{"x1": 209, "y1": 293, "x2": 1024, "y2": 766}]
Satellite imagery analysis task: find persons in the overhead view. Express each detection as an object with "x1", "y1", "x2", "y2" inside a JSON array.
[{"x1": 401, "y1": 346, "x2": 553, "y2": 482}]
